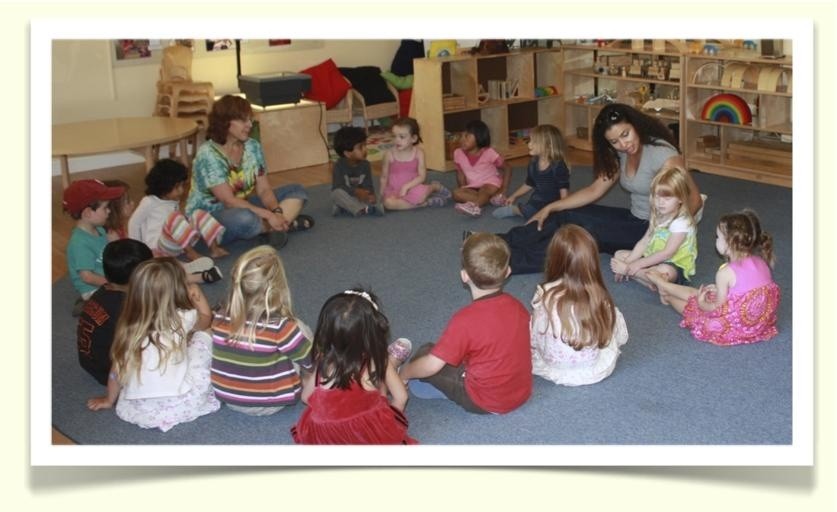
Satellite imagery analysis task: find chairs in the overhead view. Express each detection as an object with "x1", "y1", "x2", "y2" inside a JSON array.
[
  {"x1": 152, "y1": 45, "x2": 214, "y2": 168},
  {"x1": 301, "y1": 77, "x2": 353, "y2": 127},
  {"x1": 353, "y1": 81, "x2": 400, "y2": 137}
]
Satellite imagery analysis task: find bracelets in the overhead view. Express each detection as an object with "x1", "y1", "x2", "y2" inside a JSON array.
[{"x1": 272, "y1": 206, "x2": 282, "y2": 215}]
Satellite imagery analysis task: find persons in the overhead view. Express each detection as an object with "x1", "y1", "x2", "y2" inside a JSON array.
[
  {"x1": 85, "y1": 255, "x2": 223, "y2": 434},
  {"x1": 527, "y1": 223, "x2": 630, "y2": 389},
  {"x1": 396, "y1": 229, "x2": 533, "y2": 419},
  {"x1": 183, "y1": 94, "x2": 316, "y2": 251},
  {"x1": 62, "y1": 178, "x2": 222, "y2": 303},
  {"x1": 102, "y1": 179, "x2": 140, "y2": 242},
  {"x1": 377, "y1": 116, "x2": 453, "y2": 213},
  {"x1": 326, "y1": 125, "x2": 385, "y2": 219},
  {"x1": 126, "y1": 158, "x2": 233, "y2": 263},
  {"x1": 211, "y1": 243, "x2": 318, "y2": 418},
  {"x1": 461, "y1": 102, "x2": 705, "y2": 277},
  {"x1": 286, "y1": 284, "x2": 419, "y2": 444},
  {"x1": 75, "y1": 238, "x2": 156, "y2": 388},
  {"x1": 606, "y1": 163, "x2": 702, "y2": 291},
  {"x1": 644, "y1": 207, "x2": 782, "y2": 349},
  {"x1": 449, "y1": 119, "x2": 513, "y2": 220},
  {"x1": 490, "y1": 124, "x2": 571, "y2": 222}
]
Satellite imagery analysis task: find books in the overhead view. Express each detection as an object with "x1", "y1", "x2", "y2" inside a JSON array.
[{"x1": 441, "y1": 92, "x2": 468, "y2": 115}]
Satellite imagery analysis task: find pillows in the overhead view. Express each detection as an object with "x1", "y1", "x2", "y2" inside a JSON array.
[
  {"x1": 298, "y1": 58, "x2": 353, "y2": 110},
  {"x1": 337, "y1": 66, "x2": 396, "y2": 106}
]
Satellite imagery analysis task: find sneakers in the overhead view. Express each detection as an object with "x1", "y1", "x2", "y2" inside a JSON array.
[
  {"x1": 373, "y1": 204, "x2": 385, "y2": 216},
  {"x1": 490, "y1": 194, "x2": 508, "y2": 206},
  {"x1": 331, "y1": 204, "x2": 343, "y2": 217},
  {"x1": 409, "y1": 379, "x2": 447, "y2": 401},
  {"x1": 455, "y1": 201, "x2": 481, "y2": 217},
  {"x1": 183, "y1": 256, "x2": 224, "y2": 283},
  {"x1": 428, "y1": 180, "x2": 452, "y2": 206}
]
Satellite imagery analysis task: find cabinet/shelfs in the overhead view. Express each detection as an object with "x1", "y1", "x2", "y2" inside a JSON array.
[
  {"x1": 685, "y1": 49, "x2": 792, "y2": 188},
  {"x1": 564, "y1": 45, "x2": 684, "y2": 154},
  {"x1": 408, "y1": 47, "x2": 565, "y2": 173}
]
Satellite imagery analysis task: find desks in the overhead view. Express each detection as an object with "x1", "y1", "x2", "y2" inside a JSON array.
[
  {"x1": 214, "y1": 93, "x2": 330, "y2": 175},
  {"x1": 52, "y1": 117, "x2": 199, "y2": 190}
]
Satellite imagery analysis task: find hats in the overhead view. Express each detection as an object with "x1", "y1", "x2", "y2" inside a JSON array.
[{"x1": 62, "y1": 180, "x2": 124, "y2": 211}]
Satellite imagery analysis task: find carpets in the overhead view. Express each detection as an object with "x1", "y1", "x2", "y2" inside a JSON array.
[{"x1": 52, "y1": 164, "x2": 793, "y2": 445}]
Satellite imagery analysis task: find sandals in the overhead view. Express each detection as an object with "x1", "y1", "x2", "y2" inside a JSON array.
[
  {"x1": 252, "y1": 230, "x2": 287, "y2": 250},
  {"x1": 289, "y1": 215, "x2": 314, "y2": 232},
  {"x1": 388, "y1": 338, "x2": 413, "y2": 363}
]
